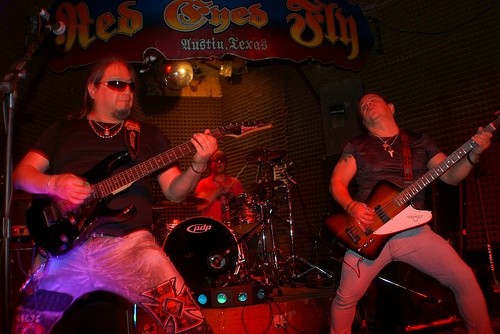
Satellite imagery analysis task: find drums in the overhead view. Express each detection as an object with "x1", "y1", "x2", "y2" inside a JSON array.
[
  {"x1": 162, "y1": 216, "x2": 245, "y2": 289},
  {"x1": 222, "y1": 191, "x2": 263, "y2": 237}
]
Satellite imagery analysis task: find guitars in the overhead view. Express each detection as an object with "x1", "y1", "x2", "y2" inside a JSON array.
[
  {"x1": 25, "y1": 120, "x2": 276, "y2": 259},
  {"x1": 322, "y1": 111, "x2": 500, "y2": 261}
]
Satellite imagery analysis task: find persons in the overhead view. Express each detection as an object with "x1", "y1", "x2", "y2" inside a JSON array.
[
  {"x1": 14, "y1": 52, "x2": 218, "y2": 334},
  {"x1": 194, "y1": 150, "x2": 255, "y2": 227},
  {"x1": 328, "y1": 91, "x2": 493, "y2": 334}
]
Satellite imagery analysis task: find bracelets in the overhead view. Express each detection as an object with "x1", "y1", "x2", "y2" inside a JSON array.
[
  {"x1": 345, "y1": 200, "x2": 354, "y2": 211},
  {"x1": 47, "y1": 174, "x2": 55, "y2": 193},
  {"x1": 190, "y1": 164, "x2": 207, "y2": 173},
  {"x1": 467, "y1": 150, "x2": 477, "y2": 167}
]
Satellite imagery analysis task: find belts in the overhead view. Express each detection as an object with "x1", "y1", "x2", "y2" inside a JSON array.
[{"x1": 89, "y1": 230, "x2": 124, "y2": 237}]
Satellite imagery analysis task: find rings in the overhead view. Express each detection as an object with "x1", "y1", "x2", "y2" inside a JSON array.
[
  {"x1": 366, "y1": 209, "x2": 370, "y2": 214},
  {"x1": 358, "y1": 222, "x2": 360, "y2": 225},
  {"x1": 361, "y1": 218, "x2": 365, "y2": 222}
]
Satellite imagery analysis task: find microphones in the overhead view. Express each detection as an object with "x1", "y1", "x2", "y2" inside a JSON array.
[
  {"x1": 37, "y1": 7, "x2": 66, "y2": 35},
  {"x1": 213, "y1": 256, "x2": 224, "y2": 267}
]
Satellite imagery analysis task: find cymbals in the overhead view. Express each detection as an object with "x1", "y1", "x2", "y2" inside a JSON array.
[
  {"x1": 245, "y1": 147, "x2": 287, "y2": 162},
  {"x1": 151, "y1": 196, "x2": 207, "y2": 208},
  {"x1": 251, "y1": 178, "x2": 282, "y2": 196}
]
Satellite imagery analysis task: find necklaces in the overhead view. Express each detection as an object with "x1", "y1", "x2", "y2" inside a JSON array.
[
  {"x1": 367, "y1": 129, "x2": 400, "y2": 157},
  {"x1": 88, "y1": 113, "x2": 124, "y2": 138}
]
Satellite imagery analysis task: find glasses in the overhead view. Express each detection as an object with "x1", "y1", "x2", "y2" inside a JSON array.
[
  {"x1": 210, "y1": 157, "x2": 227, "y2": 162},
  {"x1": 96, "y1": 79, "x2": 137, "y2": 94}
]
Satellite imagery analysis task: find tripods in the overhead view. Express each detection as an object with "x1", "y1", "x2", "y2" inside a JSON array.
[{"x1": 249, "y1": 160, "x2": 336, "y2": 297}]
